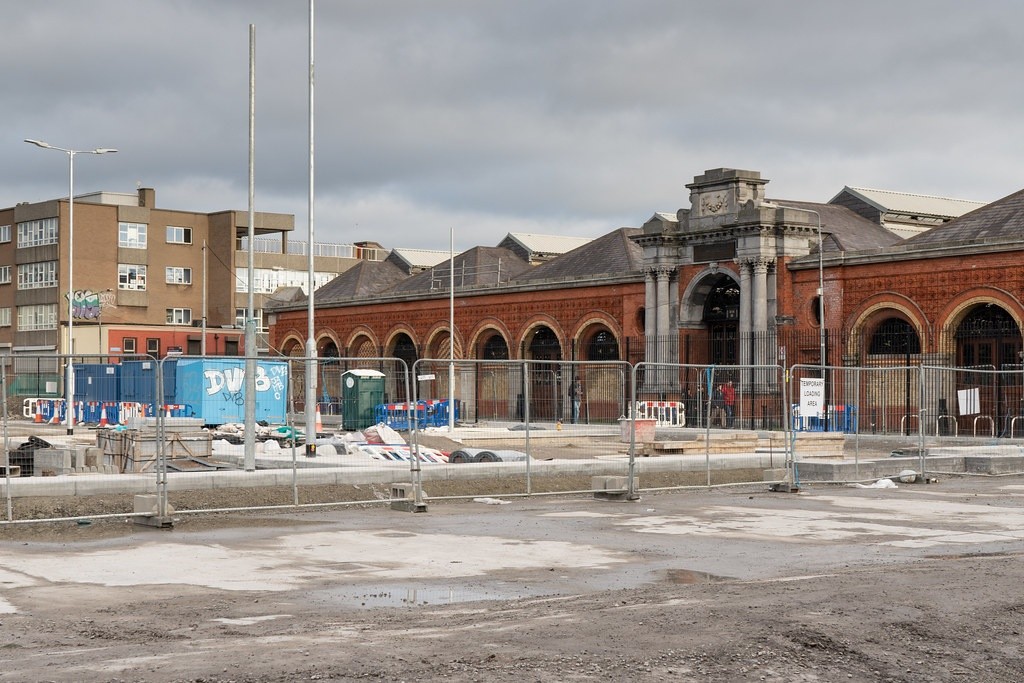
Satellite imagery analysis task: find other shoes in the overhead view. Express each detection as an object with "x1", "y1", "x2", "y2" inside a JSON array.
[
  {"x1": 687, "y1": 423, "x2": 691, "y2": 428},
  {"x1": 731, "y1": 426, "x2": 735, "y2": 429},
  {"x1": 720, "y1": 426, "x2": 729, "y2": 429}
]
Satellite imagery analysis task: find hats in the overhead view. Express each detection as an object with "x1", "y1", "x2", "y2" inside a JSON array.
[{"x1": 575, "y1": 376, "x2": 581, "y2": 382}]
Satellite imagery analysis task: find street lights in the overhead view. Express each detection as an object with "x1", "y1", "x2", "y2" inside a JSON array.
[
  {"x1": 757, "y1": 202, "x2": 825, "y2": 379},
  {"x1": 24, "y1": 139, "x2": 119, "y2": 435}
]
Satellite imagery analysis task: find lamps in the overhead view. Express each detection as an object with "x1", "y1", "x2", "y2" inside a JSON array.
[{"x1": 710, "y1": 262, "x2": 719, "y2": 274}]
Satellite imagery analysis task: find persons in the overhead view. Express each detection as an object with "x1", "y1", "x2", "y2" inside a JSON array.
[
  {"x1": 568, "y1": 376, "x2": 583, "y2": 424},
  {"x1": 711, "y1": 380, "x2": 734, "y2": 428}
]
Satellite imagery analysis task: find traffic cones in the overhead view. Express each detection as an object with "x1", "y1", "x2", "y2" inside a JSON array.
[
  {"x1": 97, "y1": 403, "x2": 108, "y2": 427},
  {"x1": 166, "y1": 407, "x2": 171, "y2": 417},
  {"x1": 73, "y1": 402, "x2": 76, "y2": 425},
  {"x1": 32, "y1": 402, "x2": 45, "y2": 423},
  {"x1": 50, "y1": 404, "x2": 61, "y2": 424},
  {"x1": 316, "y1": 405, "x2": 323, "y2": 433}
]
